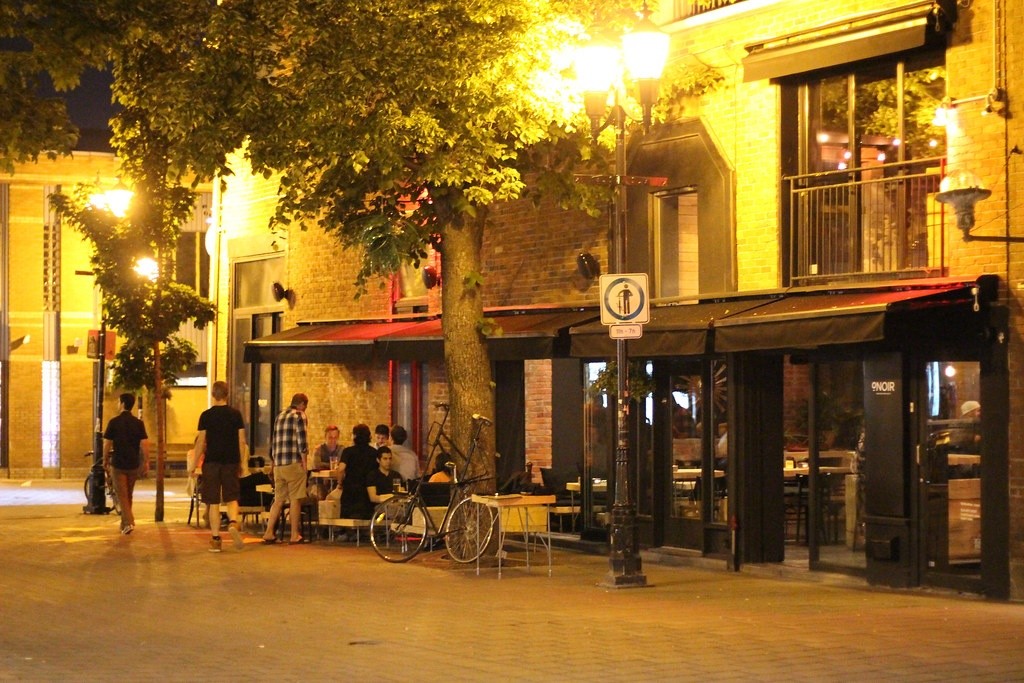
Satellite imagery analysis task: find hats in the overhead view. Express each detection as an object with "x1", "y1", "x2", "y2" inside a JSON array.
[{"x1": 961, "y1": 401, "x2": 980, "y2": 416}]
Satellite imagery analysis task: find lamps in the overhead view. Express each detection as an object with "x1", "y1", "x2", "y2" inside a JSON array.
[
  {"x1": 578, "y1": 253, "x2": 599, "y2": 279},
  {"x1": 936, "y1": 170, "x2": 1024, "y2": 244},
  {"x1": 422, "y1": 265, "x2": 440, "y2": 288},
  {"x1": 272, "y1": 282, "x2": 288, "y2": 302}
]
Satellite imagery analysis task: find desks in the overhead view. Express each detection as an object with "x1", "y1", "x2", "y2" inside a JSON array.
[
  {"x1": 566, "y1": 482, "x2": 607, "y2": 533},
  {"x1": 474, "y1": 494, "x2": 556, "y2": 578},
  {"x1": 309, "y1": 470, "x2": 343, "y2": 539},
  {"x1": 784, "y1": 466, "x2": 850, "y2": 541},
  {"x1": 672, "y1": 468, "x2": 724, "y2": 518}
]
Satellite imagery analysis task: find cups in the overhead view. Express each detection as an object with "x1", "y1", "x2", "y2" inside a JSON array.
[
  {"x1": 329, "y1": 456, "x2": 338, "y2": 470},
  {"x1": 392, "y1": 478, "x2": 401, "y2": 493}
]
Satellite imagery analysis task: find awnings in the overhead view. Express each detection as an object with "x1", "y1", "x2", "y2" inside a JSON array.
[{"x1": 243, "y1": 287, "x2": 965, "y2": 365}]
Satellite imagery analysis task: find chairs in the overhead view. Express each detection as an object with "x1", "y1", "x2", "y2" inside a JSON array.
[
  {"x1": 270, "y1": 475, "x2": 313, "y2": 542},
  {"x1": 187, "y1": 479, "x2": 206, "y2": 526},
  {"x1": 784, "y1": 473, "x2": 846, "y2": 546}
]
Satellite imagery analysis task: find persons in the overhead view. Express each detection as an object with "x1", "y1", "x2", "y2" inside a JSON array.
[
  {"x1": 186, "y1": 435, "x2": 205, "y2": 497},
  {"x1": 189, "y1": 381, "x2": 246, "y2": 552},
  {"x1": 313, "y1": 425, "x2": 346, "y2": 468},
  {"x1": 365, "y1": 446, "x2": 402, "y2": 519},
  {"x1": 337, "y1": 424, "x2": 378, "y2": 541},
  {"x1": 103, "y1": 393, "x2": 149, "y2": 534},
  {"x1": 369, "y1": 424, "x2": 390, "y2": 449},
  {"x1": 387, "y1": 425, "x2": 422, "y2": 482},
  {"x1": 429, "y1": 453, "x2": 452, "y2": 482},
  {"x1": 261, "y1": 393, "x2": 310, "y2": 545}
]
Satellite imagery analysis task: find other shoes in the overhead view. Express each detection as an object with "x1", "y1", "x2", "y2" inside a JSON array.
[
  {"x1": 202, "y1": 512, "x2": 210, "y2": 528},
  {"x1": 208, "y1": 539, "x2": 222, "y2": 553},
  {"x1": 221, "y1": 518, "x2": 229, "y2": 528},
  {"x1": 228, "y1": 521, "x2": 245, "y2": 549},
  {"x1": 122, "y1": 525, "x2": 134, "y2": 534}
]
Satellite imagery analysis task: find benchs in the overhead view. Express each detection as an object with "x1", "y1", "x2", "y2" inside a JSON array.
[
  {"x1": 527, "y1": 465, "x2": 608, "y2": 533},
  {"x1": 239, "y1": 480, "x2": 462, "y2": 552}
]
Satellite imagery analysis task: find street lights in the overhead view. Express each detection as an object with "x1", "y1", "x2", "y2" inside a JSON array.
[
  {"x1": 80, "y1": 174, "x2": 161, "y2": 514},
  {"x1": 582, "y1": 28, "x2": 670, "y2": 590}
]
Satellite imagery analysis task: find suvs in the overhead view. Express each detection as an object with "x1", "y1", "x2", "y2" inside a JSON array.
[
  {"x1": 920, "y1": 428, "x2": 983, "y2": 479},
  {"x1": 851, "y1": 417, "x2": 982, "y2": 475}
]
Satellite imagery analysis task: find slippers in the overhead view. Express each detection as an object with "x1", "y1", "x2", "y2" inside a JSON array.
[
  {"x1": 288, "y1": 536, "x2": 311, "y2": 544},
  {"x1": 261, "y1": 536, "x2": 283, "y2": 544}
]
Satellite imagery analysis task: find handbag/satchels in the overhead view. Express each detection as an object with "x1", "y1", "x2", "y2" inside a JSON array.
[{"x1": 376, "y1": 497, "x2": 411, "y2": 524}]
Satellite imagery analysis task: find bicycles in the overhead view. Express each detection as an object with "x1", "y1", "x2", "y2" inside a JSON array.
[
  {"x1": 83, "y1": 448, "x2": 122, "y2": 516},
  {"x1": 369, "y1": 397, "x2": 500, "y2": 563}
]
